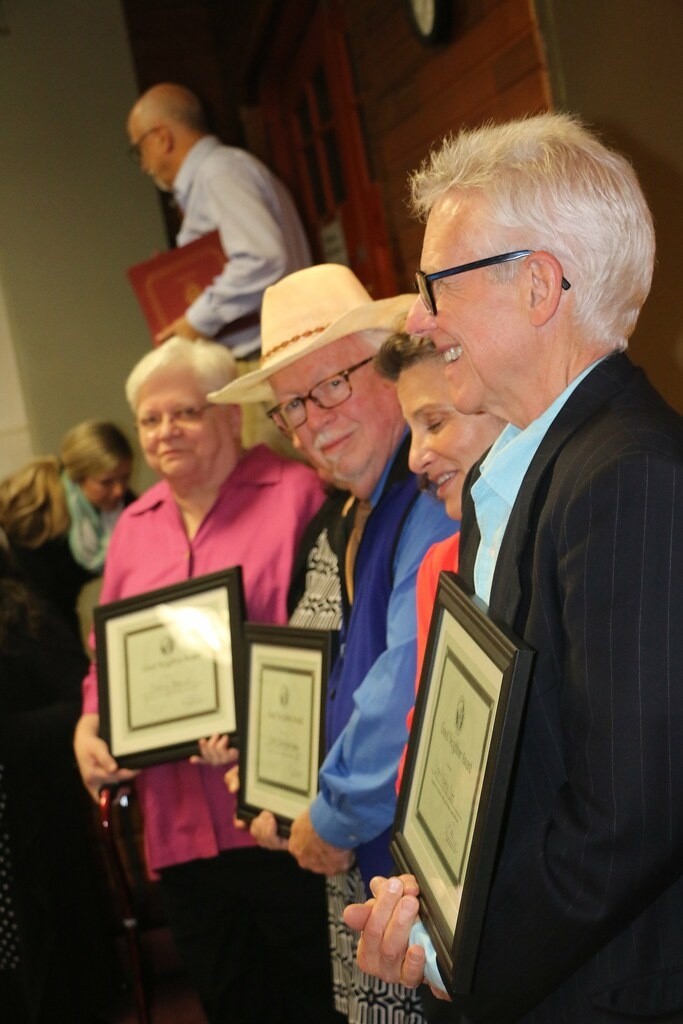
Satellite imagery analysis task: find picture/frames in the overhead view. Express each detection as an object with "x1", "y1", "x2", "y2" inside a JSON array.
[
  {"x1": 227, "y1": 618, "x2": 340, "y2": 845},
  {"x1": 381, "y1": 568, "x2": 534, "y2": 997},
  {"x1": 92, "y1": 561, "x2": 249, "y2": 777}
]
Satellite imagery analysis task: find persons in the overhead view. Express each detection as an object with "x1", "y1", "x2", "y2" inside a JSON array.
[
  {"x1": 200, "y1": 473, "x2": 375, "y2": 1024},
  {"x1": 370, "y1": 307, "x2": 513, "y2": 804},
  {"x1": 341, "y1": 108, "x2": 683, "y2": 1024},
  {"x1": 73, "y1": 334, "x2": 347, "y2": 1023},
  {"x1": 126, "y1": 81, "x2": 314, "y2": 468},
  {"x1": 223, "y1": 261, "x2": 464, "y2": 1023},
  {"x1": 0, "y1": 418, "x2": 143, "y2": 1022}
]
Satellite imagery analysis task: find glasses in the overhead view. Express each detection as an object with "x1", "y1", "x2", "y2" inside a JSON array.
[
  {"x1": 132, "y1": 398, "x2": 234, "y2": 431},
  {"x1": 125, "y1": 125, "x2": 166, "y2": 167},
  {"x1": 413, "y1": 247, "x2": 570, "y2": 318},
  {"x1": 264, "y1": 355, "x2": 396, "y2": 435}
]
